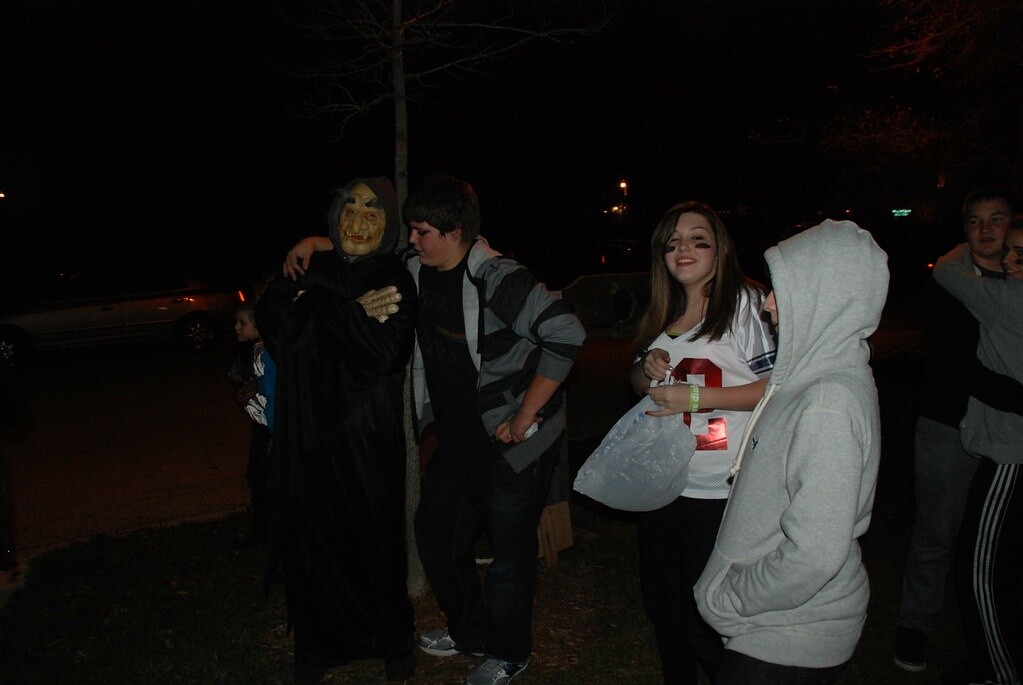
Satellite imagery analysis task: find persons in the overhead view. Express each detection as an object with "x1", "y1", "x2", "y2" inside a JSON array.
[
  {"x1": 692, "y1": 214, "x2": 889, "y2": 682},
  {"x1": 249, "y1": 175, "x2": 420, "y2": 685},
  {"x1": 622, "y1": 200, "x2": 782, "y2": 684},
  {"x1": 884, "y1": 180, "x2": 1023, "y2": 672},
  {"x1": 231, "y1": 307, "x2": 280, "y2": 507},
  {"x1": 285, "y1": 176, "x2": 586, "y2": 685},
  {"x1": 928, "y1": 223, "x2": 1023, "y2": 685}
]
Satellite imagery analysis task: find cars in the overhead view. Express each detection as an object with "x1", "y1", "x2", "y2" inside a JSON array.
[
  {"x1": 0, "y1": 258, "x2": 236, "y2": 371},
  {"x1": 557, "y1": 270, "x2": 652, "y2": 393}
]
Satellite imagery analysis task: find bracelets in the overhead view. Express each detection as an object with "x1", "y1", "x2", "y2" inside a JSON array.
[{"x1": 688, "y1": 384, "x2": 700, "y2": 412}]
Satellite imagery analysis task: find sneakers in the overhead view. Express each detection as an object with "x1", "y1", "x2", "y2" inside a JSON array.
[
  {"x1": 256, "y1": 619, "x2": 295, "y2": 647},
  {"x1": 893, "y1": 626, "x2": 929, "y2": 672},
  {"x1": 465, "y1": 657, "x2": 530, "y2": 685},
  {"x1": 415, "y1": 626, "x2": 486, "y2": 657}
]
